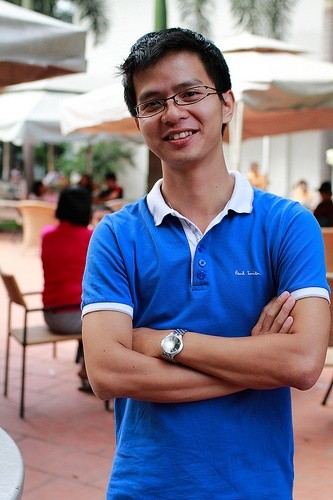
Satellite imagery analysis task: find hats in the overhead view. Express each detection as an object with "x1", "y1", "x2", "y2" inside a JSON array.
[{"x1": 319, "y1": 182, "x2": 332, "y2": 194}]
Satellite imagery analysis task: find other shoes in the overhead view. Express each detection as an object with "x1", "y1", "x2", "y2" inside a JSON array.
[{"x1": 79, "y1": 383, "x2": 94, "y2": 401}]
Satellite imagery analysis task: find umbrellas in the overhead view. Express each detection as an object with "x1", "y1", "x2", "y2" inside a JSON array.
[{"x1": 1, "y1": 2, "x2": 333, "y2": 196}]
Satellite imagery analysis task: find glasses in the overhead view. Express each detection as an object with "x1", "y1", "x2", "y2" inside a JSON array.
[{"x1": 132, "y1": 85, "x2": 220, "y2": 118}]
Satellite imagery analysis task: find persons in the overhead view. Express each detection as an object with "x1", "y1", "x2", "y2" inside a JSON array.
[
  {"x1": 78, "y1": 28, "x2": 330, "y2": 500},
  {"x1": 245, "y1": 161, "x2": 332, "y2": 226},
  {"x1": 39, "y1": 186, "x2": 102, "y2": 393},
  {"x1": 0, "y1": 168, "x2": 123, "y2": 212}
]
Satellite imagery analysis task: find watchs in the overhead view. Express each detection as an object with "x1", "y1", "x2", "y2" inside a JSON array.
[{"x1": 159, "y1": 328, "x2": 186, "y2": 361}]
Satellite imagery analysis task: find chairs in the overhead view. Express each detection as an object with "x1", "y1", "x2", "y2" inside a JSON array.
[
  {"x1": 15, "y1": 200, "x2": 59, "y2": 249},
  {"x1": 0, "y1": 269, "x2": 115, "y2": 418}
]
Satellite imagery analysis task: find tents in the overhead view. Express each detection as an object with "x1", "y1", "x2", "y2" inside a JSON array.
[{"x1": 2, "y1": 91, "x2": 109, "y2": 197}]
